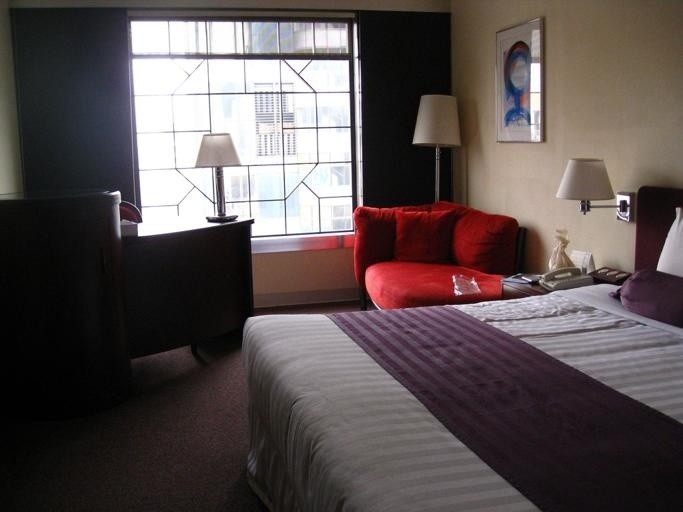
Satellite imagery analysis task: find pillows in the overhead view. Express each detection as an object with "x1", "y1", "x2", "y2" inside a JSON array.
[
  {"x1": 616, "y1": 270, "x2": 683, "y2": 329},
  {"x1": 655, "y1": 207, "x2": 683, "y2": 281},
  {"x1": 393, "y1": 207, "x2": 455, "y2": 265}
]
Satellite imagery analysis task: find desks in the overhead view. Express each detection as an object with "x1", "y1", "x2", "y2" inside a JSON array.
[{"x1": 0, "y1": 184, "x2": 257, "y2": 396}]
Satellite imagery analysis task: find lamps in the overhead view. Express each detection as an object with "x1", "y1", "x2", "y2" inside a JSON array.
[
  {"x1": 196, "y1": 133, "x2": 243, "y2": 222},
  {"x1": 554, "y1": 160, "x2": 635, "y2": 227},
  {"x1": 409, "y1": 94, "x2": 463, "y2": 201}
]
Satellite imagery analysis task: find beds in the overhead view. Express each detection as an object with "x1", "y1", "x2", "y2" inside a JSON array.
[{"x1": 247, "y1": 182, "x2": 683, "y2": 512}]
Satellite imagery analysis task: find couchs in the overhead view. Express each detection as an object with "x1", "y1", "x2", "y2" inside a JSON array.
[{"x1": 340, "y1": 198, "x2": 525, "y2": 308}]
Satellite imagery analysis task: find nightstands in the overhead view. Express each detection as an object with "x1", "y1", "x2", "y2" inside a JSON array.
[{"x1": 500, "y1": 271, "x2": 549, "y2": 300}]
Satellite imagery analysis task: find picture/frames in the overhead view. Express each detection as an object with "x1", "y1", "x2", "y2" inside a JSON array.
[{"x1": 494, "y1": 17, "x2": 548, "y2": 148}]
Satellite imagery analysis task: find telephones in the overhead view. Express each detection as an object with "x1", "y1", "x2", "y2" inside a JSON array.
[{"x1": 539, "y1": 268, "x2": 593, "y2": 291}]
